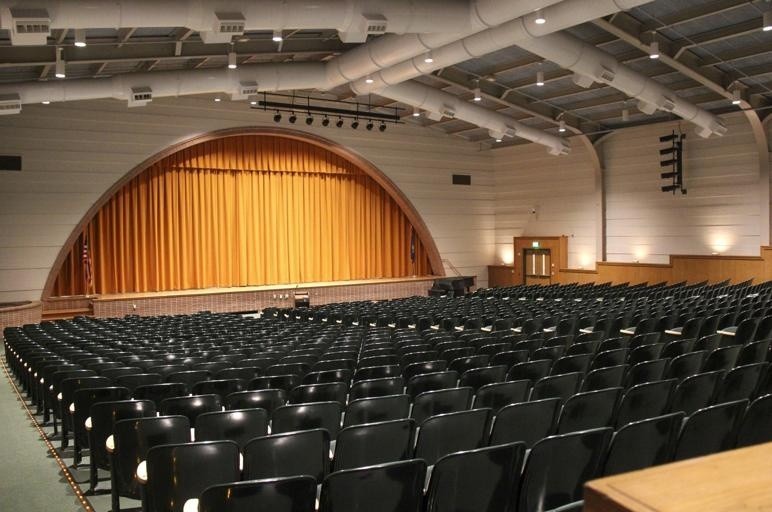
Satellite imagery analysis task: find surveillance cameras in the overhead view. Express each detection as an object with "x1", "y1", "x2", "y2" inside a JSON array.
[{"x1": 532, "y1": 209, "x2": 536, "y2": 213}]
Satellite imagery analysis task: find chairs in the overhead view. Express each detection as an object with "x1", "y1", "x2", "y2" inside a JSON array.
[{"x1": 3, "y1": 278, "x2": 772, "y2": 512}]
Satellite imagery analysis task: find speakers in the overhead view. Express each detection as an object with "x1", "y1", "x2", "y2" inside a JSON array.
[
  {"x1": 453, "y1": 175, "x2": 470, "y2": 184},
  {"x1": 0, "y1": 156, "x2": 21, "y2": 170}
]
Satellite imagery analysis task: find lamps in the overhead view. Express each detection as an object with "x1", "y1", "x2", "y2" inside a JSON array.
[
  {"x1": 424, "y1": 52, "x2": 433, "y2": 63},
  {"x1": 474, "y1": 88, "x2": 482, "y2": 101},
  {"x1": 272, "y1": 29, "x2": 282, "y2": 41},
  {"x1": 732, "y1": 89, "x2": 741, "y2": 105},
  {"x1": 75, "y1": 28, "x2": 87, "y2": 48},
  {"x1": 537, "y1": 71, "x2": 545, "y2": 86},
  {"x1": 250, "y1": 93, "x2": 405, "y2": 132},
  {"x1": 365, "y1": 75, "x2": 374, "y2": 84},
  {"x1": 228, "y1": 41, "x2": 237, "y2": 69},
  {"x1": 659, "y1": 125, "x2": 687, "y2": 195},
  {"x1": 650, "y1": 42, "x2": 660, "y2": 59},
  {"x1": 55, "y1": 47, "x2": 66, "y2": 78},
  {"x1": 535, "y1": 9, "x2": 546, "y2": 25},
  {"x1": 412, "y1": 106, "x2": 420, "y2": 117},
  {"x1": 763, "y1": 11, "x2": 772, "y2": 32},
  {"x1": 622, "y1": 109, "x2": 630, "y2": 122},
  {"x1": 559, "y1": 120, "x2": 566, "y2": 132}
]
